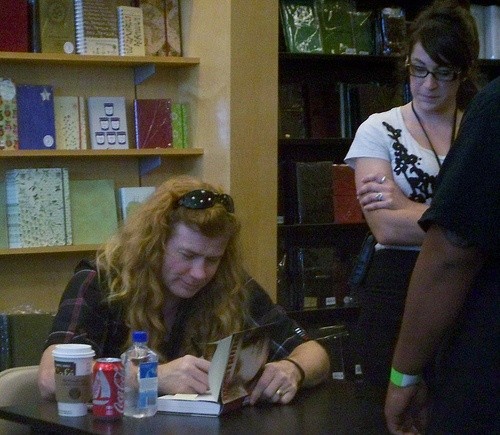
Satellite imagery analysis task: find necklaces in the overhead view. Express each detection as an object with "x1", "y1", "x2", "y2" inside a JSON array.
[{"x1": 411, "y1": 100, "x2": 458, "y2": 167}]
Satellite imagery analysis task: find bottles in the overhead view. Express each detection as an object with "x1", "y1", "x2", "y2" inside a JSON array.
[{"x1": 120, "y1": 330, "x2": 159, "y2": 418}]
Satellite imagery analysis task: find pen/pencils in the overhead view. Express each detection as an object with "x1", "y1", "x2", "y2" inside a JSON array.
[{"x1": 189, "y1": 336, "x2": 204, "y2": 359}]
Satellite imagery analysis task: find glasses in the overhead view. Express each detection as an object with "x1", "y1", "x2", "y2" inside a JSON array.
[
  {"x1": 173, "y1": 190, "x2": 235, "y2": 213},
  {"x1": 404, "y1": 56, "x2": 464, "y2": 82}
]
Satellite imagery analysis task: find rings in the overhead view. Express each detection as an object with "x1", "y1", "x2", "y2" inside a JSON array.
[
  {"x1": 379, "y1": 176, "x2": 387, "y2": 183},
  {"x1": 276, "y1": 389, "x2": 284, "y2": 396},
  {"x1": 376, "y1": 193, "x2": 381, "y2": 200}
]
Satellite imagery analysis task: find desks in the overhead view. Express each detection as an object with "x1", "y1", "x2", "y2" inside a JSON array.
[{"x1": 1, "y1": 378, "x2": 388, "y2": 435}]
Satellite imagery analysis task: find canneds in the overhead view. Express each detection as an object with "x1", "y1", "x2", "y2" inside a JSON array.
[{"x1": 92, "y1": 358, "x2": 125, "y2": 418}]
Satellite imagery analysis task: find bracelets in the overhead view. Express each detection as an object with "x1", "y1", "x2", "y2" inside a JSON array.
[
  {"x1": 282, "y1": 358, "x2": 306, "y2": 386},
  {"x1": 389, "y1": 366, "x2": 425, "y2": 386}
]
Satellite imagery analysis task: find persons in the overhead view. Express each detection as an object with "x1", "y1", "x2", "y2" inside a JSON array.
[
  {"x1": 384, "y1": 78, "x2": 500, "y2": 435},
  {"x1": 344, "y1": 0, "x2": 483, "y2": 397},
  {"x1": 36, "y1": 176, "x2": 331, "y2": 405}
]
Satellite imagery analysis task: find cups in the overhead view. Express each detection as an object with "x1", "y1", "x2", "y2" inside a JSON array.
[{"x1": 50, "y1": 344, "x2": 96, "y2": 417}]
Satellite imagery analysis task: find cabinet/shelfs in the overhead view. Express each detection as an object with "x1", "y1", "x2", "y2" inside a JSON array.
[
  {"x1": 0, "y1": 1, "x2": 199, "y2": 255},
  {"x1": 229, "y1": 0, "x2": 500, "y2": 339}
]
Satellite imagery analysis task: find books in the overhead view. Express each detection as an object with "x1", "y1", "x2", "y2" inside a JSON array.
[
  {"x1": 0, "y1": 169, "x2": 156, "y2": 370},
  {"x1": 276, "y1": 0, "x2": 500, "y2": 308},
  {"x1": 0, "y1": 0, "x2": 184, "y2": 58},
  {"x1": 0, "y1": 79, "x2": 190, "y2": 151},
  {"x1": 153, "y1": 321, "x2": 278, "y2": 419}
]
